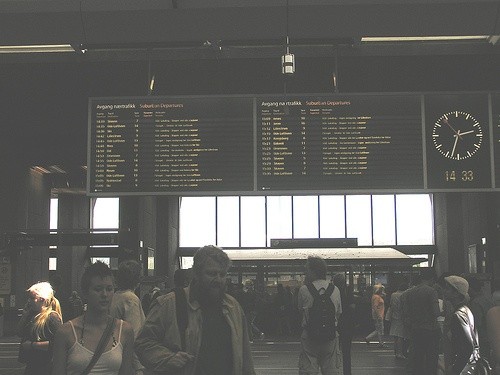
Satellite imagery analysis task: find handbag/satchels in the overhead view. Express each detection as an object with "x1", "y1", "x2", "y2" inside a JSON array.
[
  {"x1": 18, "y1": 312, "x2": 64, "y2": 366},
  {"x1": 460, "y1": 364, "x2": 487, "y2": 375}
]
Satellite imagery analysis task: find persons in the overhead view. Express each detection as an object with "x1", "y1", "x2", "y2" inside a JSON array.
[
  {"x1": 0, "y1": 267, "x2": 500, "y2": 375},
  {"x1": 53, "y1": 261, "x2": 134, "y2": 375},
  {"x1": 110, "y1": 261, "x2": 146, "y2": 375},
  {"x1": 363, "y1": 284, "x2": 390, "y2": 348},
  {"x1": 297, "y1": 256, "x2": 342, "y2": 375},
  {"x1": 15, "y1": 281, "x2": 63, "y2": 375},
  {"x1": 443, "y1": 275, "x2": 480, "y2": 375},
  {"x1": 405, "y1": 267, "x2": 440, "y2": 375},
  {"x1": 134, "y1": 245, "x2": 256, "y2": 374}
]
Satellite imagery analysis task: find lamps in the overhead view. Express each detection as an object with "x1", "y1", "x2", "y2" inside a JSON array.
[{"x1": 280, "y1": 0, "x2": 296, "y2": 79}]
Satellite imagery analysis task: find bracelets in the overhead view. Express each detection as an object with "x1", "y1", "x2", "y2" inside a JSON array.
[{"x1": 30, "y1": 341, "x2": 34, "y2": 347}]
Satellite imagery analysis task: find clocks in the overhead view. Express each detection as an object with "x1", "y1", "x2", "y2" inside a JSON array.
[{"x1": 432, "y1": 111, "x2": 484, "y2": 161}]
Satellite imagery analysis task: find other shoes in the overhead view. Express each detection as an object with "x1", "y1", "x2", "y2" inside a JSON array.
[
  {"x1": 396, "y1": 354, "x2": 408, "y2": 360},
  {"x1": 377, "y1": 343, "x2": 391, "y2": 349},
  {"x1": 363, "y1": 336, "x2": 370, "y2": 345},
  {"x1": 259, "y1": 332, "x2": 266, "y2": 341},
  {"x1": 247, "y1": 340, "x2": 256, "y2": 344}
]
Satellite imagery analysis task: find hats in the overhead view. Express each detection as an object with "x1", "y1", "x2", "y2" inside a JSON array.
[{"x1": 443, "y1": 276, "x2": 470, "y2": 296}]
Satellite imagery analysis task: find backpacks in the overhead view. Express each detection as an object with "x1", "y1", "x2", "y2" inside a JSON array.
[{"x1": 304, "y1": 282, "x2": 336, "y2": 342}]
